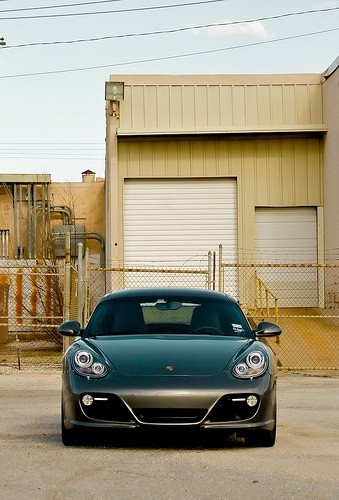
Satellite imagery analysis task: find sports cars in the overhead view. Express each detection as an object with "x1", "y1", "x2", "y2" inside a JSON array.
[{"x1": 56, "y1": 287, "x2": 282, "y2": 449}]
